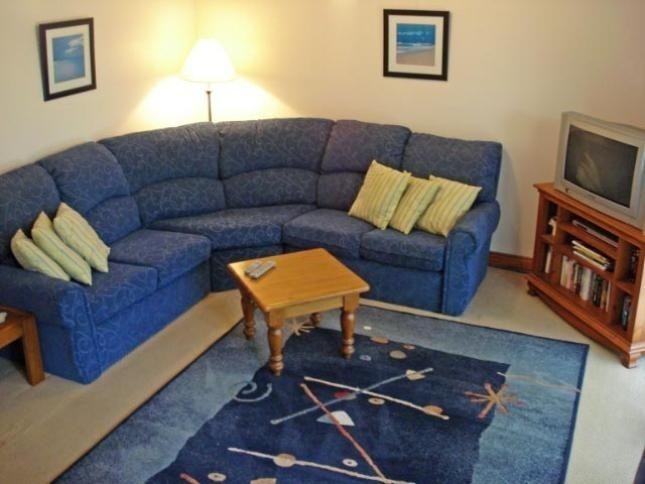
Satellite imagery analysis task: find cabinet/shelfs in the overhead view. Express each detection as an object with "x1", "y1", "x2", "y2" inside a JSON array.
[{"x1": 523, "y1": 181, "x2": 645, "y2": 369}]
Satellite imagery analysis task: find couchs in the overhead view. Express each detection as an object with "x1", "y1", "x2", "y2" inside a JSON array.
[{"x1": 0, "y1": 115, "x2": 505, "y2": 387}]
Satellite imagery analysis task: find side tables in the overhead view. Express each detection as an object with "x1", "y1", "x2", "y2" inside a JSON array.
[{"x1": 0, "y1": 303, "x2": 44, "y2": 386}]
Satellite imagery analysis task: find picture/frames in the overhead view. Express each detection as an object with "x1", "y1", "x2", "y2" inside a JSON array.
[
  {"x1": 382, "y1": 9, "x2": 451, "y2": 81},
  {"x1": 38, "y1": 16, "x2": 97, "y2": 102}
]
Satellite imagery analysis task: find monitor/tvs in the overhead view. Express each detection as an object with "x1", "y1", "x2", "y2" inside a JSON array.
[{"x1": 552, "y1": 111, "x2": 645, "y2": 232}]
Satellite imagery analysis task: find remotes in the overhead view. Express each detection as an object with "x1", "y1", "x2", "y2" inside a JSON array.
[
  {"x1": 244, "y1": 261, "x2": 260, "y2": 273},
  {"x1": 250, "y1": 260, "x2": 274, "y2": 280}
]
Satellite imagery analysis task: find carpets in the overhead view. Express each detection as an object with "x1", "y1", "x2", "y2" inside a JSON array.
[{"x1": 42, "y1": 301, "x2": 591, "y2": 482}]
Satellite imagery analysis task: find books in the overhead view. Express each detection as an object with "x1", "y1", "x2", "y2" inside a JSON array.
[
  {"x1": 545, "y1": 217, "x2": 612, "y2": 312},
  {"x1": 622, "y1": 296, "x2": 632, "y2": 328}
]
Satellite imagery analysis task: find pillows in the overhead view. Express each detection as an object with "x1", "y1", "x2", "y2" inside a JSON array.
[
  {"x1": 9, "y1": 227, "x2": 73, "y2": 283},
  {"x1": 388, "y1": 168, "x2": 440, "y2": 236},
  {"x1": 50, "y1": 200, "x2": 112, "y2": 273},
  {"x1": 415, "y1": 173, "x2": 483, "y2": 238},
  {"x1": 346, "y1": 158, "x2": 412, "y2": 230},
  {"x1": 30, "y1": 209, "x2": 93, "y2": 287}
]
